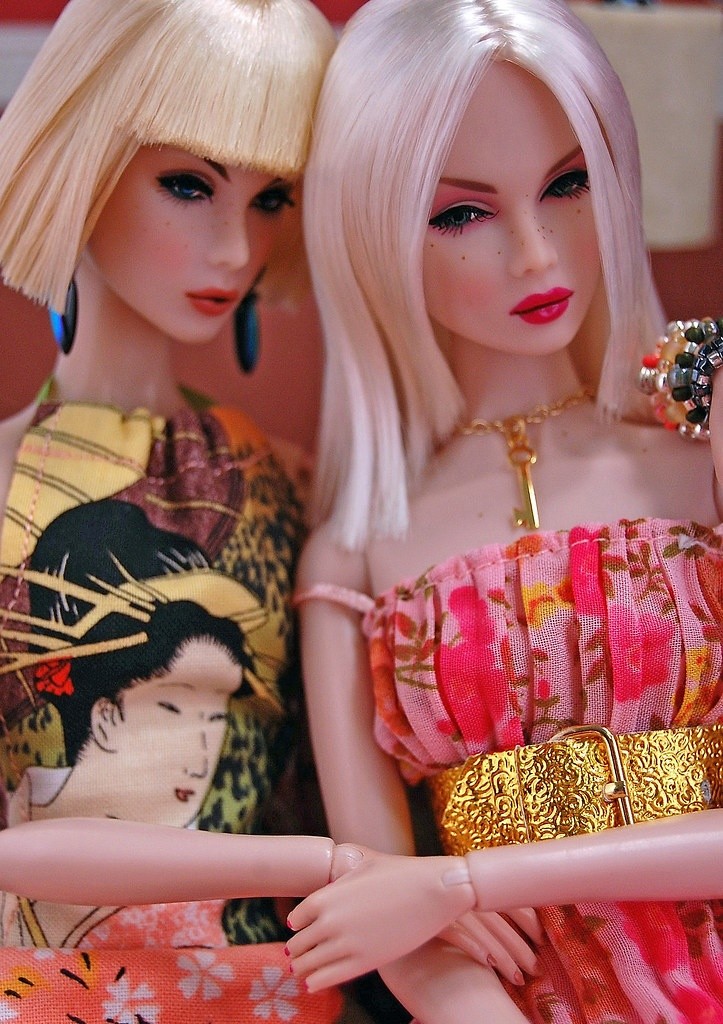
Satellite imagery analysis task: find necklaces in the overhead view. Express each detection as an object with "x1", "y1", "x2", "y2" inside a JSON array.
[{"x1": 457, "y1": 382, "x2": 595, "y2": 529}]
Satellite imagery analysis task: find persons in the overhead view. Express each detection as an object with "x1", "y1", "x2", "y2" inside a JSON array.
[
  {"x1": 0, "y1": 1, "x2": 543, "y2": 1024},
  {"x1": 286, "y1": 0, "x2": 723, "y2": 1024}
]
